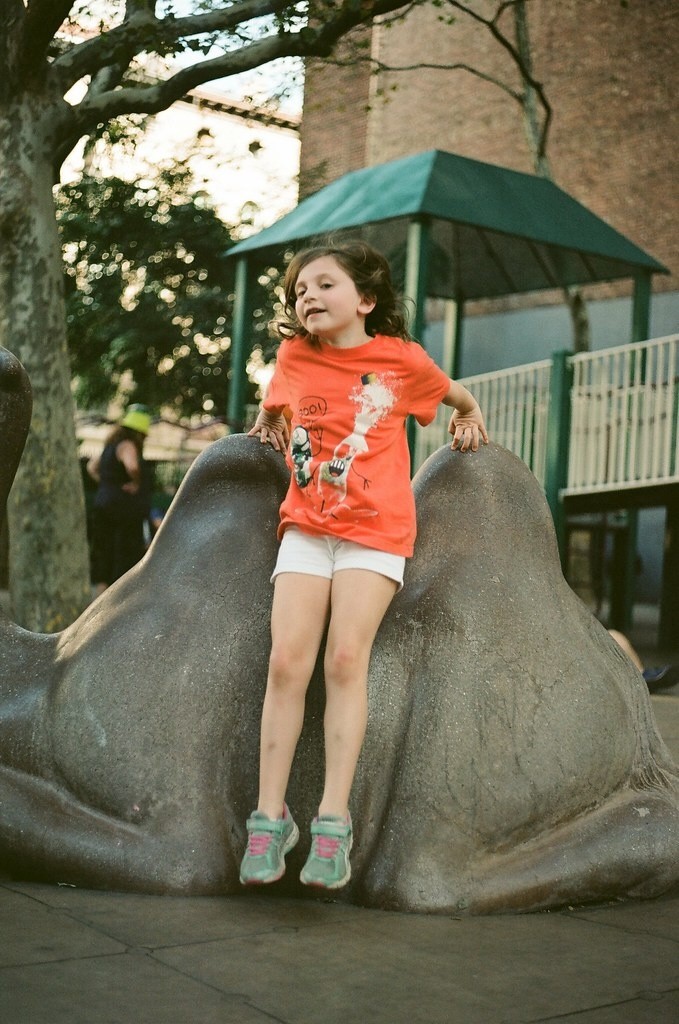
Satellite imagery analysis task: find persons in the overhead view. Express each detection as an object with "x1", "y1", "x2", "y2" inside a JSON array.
[
  {"x1": 608, "y1": 629, "x2": 679, "y2": 695},
  {"x1": 87, "y1": 406, "x2": 153, "y2": 598},
  {"x1": 238, "y1": 242, "x2": 489, "y2": 890}
]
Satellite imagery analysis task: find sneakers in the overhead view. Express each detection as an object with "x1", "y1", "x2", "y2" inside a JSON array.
[
  {"x1": 299, "y1": 808, "x2": 355, "y2": 891},
  {"x1": 239, "y1": 802, "x2": 300, "y2": 886}
]
